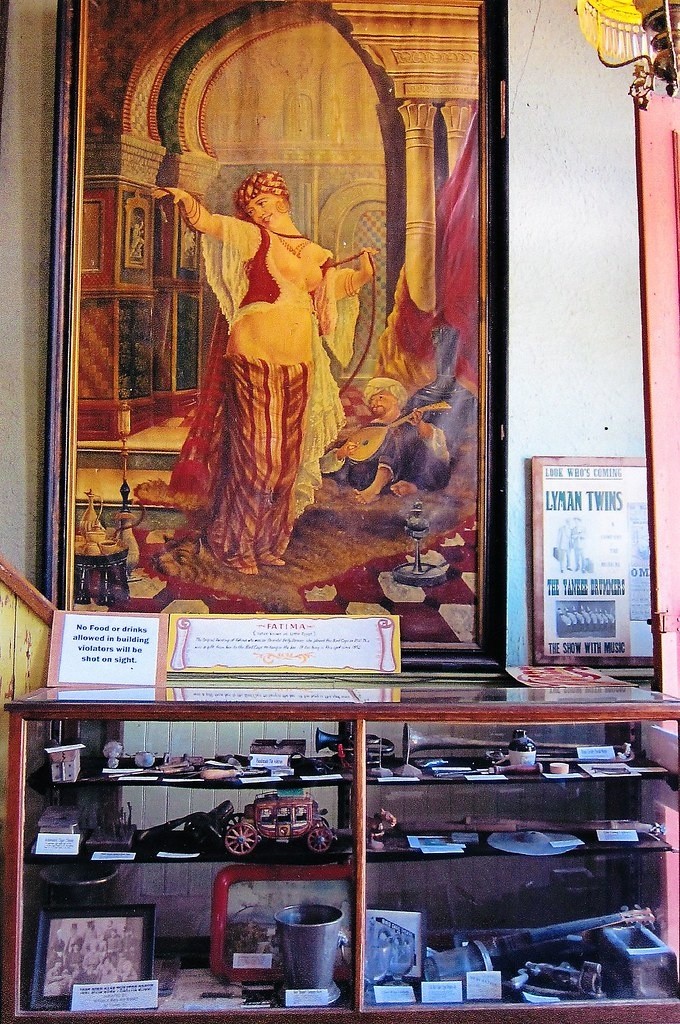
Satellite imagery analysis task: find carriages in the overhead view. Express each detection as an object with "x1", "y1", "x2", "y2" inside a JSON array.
[{"x1": 221, "y1": 790, "x2": 398, "y2": 857}]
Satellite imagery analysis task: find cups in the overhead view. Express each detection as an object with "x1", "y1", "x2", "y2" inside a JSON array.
[
  {"x1": 424, "y1": 940, "x2": 493, "y2": 982},
  {"x1": 274, "y1": 904, "x2": 345, "y2": 989}
]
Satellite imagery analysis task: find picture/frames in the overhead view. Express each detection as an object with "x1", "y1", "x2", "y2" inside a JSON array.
[
  {"x1": 530, "y1": 455, "x2": 654, "y2": 667},
  {"x1": 209, "y1": 863, "x2": 355, "y2": 981},
  {"x1": 29, "y1": 903, "x2": 156, "y2": 1009},
  {"x1": 43, "y1": 0, "x2": 510, "y2": 680}
]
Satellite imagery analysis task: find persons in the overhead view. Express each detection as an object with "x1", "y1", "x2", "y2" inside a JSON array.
[
  {"x1": 42, "y1": 919, "x2": 141, "y2": 996},
  {"x1": 556, "y1": 605, "x2": 616, "y2": 634}
]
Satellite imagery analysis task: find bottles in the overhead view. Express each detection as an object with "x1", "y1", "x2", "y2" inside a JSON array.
[{"x1": 508, "y1": 730, "x2": 537, "y2": 765}]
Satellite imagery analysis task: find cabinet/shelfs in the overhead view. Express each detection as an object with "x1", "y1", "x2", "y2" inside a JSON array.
[{"x1": 2, "y1": 684, "x2": 680, "y2": 1024}]
